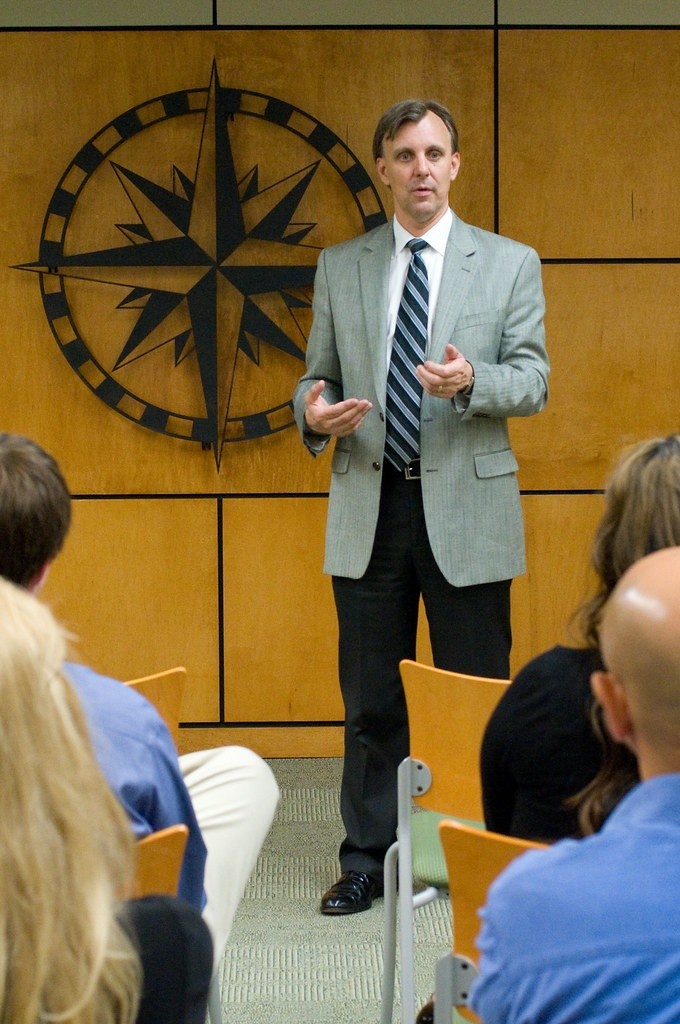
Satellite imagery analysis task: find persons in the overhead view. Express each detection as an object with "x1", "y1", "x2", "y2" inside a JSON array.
[
  {"x1": 467, "y1": 546, "x2": 680, "y2": 1024},
  {"x1": 416, "y1": 430, "x2": 680, "y2": 1024},
  {"x1": 0, "y1": 433, "x2": 280, "y2": 979},
  {"x1": 295, "y1": 99, "x2": 550, "y2": 917},
  {"x1": 0, "y1": 580, "x2": 215, "y2": 1024}
]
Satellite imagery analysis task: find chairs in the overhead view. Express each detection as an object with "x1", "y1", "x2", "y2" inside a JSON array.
[{"x1": 370, "y1": 659, "x2": 550, "y2": 1024}]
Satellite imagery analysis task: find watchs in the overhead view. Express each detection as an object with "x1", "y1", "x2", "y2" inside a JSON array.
[{"x1": 459, "y1": 377, "x2": 475, "y2": 395}]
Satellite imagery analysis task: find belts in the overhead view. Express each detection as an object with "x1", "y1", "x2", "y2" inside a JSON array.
[{"x1": 382, "y1": 455, "x2": 421, "y2": 480}]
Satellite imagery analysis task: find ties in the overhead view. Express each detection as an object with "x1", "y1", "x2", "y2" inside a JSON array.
[{"x1": 384, "y1": 238, "x2": 429, "y2": 472}]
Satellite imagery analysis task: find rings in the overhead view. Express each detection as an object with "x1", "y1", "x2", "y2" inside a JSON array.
[{"x1": 438, "y1": 386, "x2": 442, "y2": 393}]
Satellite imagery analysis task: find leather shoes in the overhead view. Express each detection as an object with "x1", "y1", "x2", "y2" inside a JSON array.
[{"x1": 321, "y1": 871, "x2": 399, "y2": 913}]
